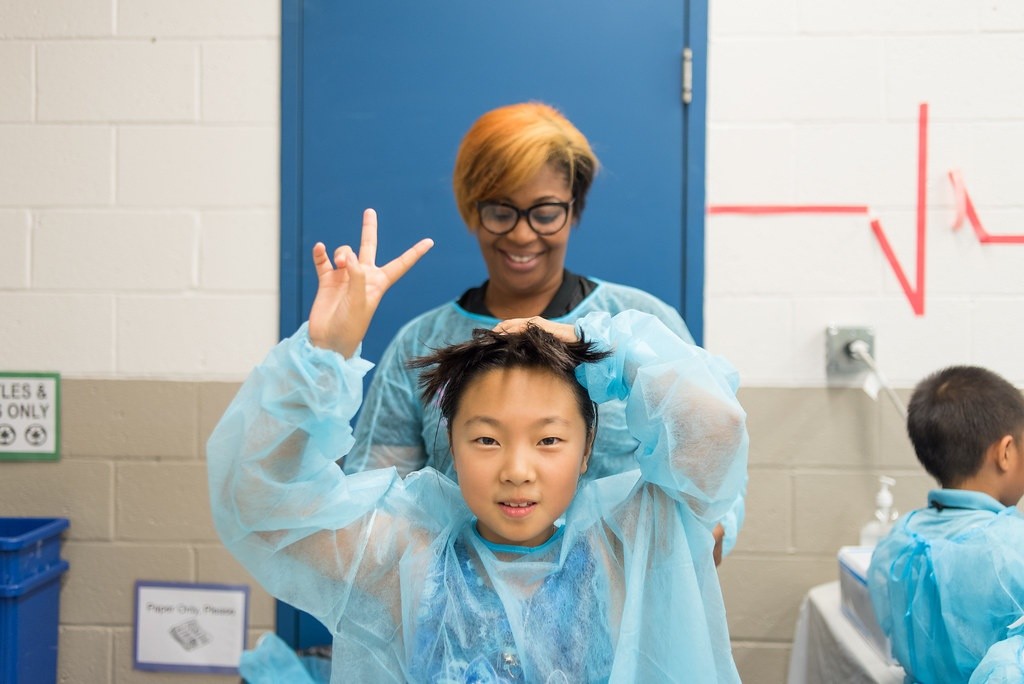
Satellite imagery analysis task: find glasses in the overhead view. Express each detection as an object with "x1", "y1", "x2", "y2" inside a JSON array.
[{"x1": 475, "y1": 198, "x2": 575, "y2": 236}]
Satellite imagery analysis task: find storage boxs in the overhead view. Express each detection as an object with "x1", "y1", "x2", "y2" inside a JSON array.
[
  {"x1": 834, "y1": 538, "x2": 894, "y2": 652},
  {"x1": 0, "y1": 517, "x2": 71, "y2": 684}
]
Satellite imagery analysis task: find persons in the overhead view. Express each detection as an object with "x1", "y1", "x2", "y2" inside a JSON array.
[
  {"x1": 326, "y1": 103, "x2": 742, "y2": 566},
  {"x1": 202, "y1": 206, "x2": 749, "y2": 684},
  {"x1": 865, "y1": 366, "x2": 1024, "y2": 684}
]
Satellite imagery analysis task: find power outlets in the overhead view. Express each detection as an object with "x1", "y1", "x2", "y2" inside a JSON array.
[{"x1": 822, "y1": 324, "x2": 874, "y2": 379}]
